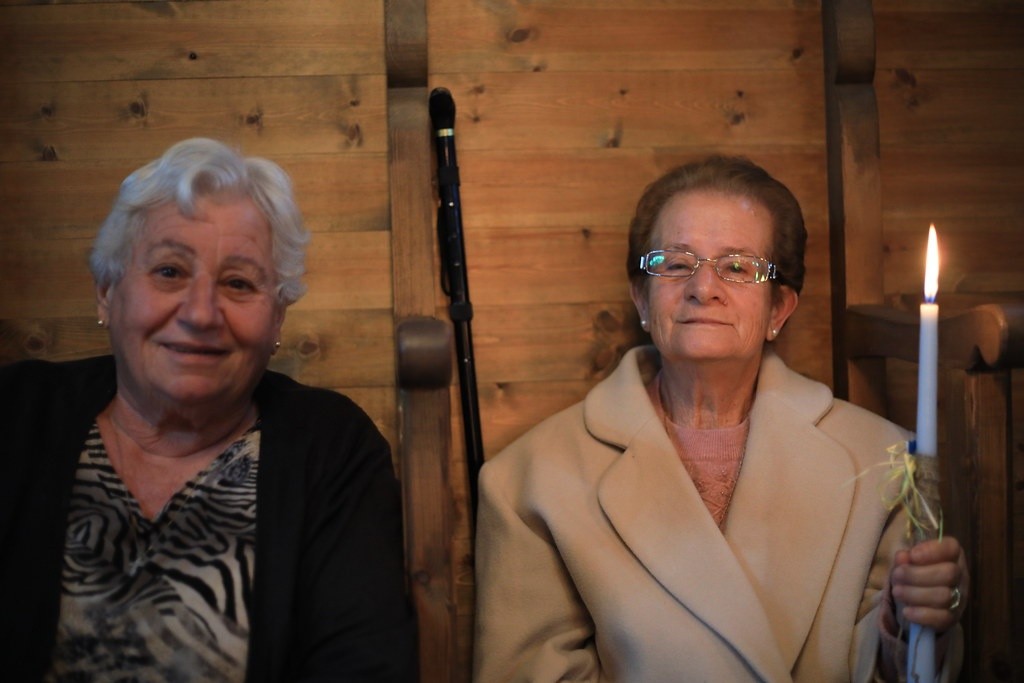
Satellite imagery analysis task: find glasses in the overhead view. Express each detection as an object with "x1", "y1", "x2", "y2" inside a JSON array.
[{"x1": 639, "y1": 250, "x2": 776, "y2": 283}]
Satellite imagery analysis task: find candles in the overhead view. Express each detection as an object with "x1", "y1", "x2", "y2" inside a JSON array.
[{"x1": 915, "y1": 223, "x2": 940, "y2": 461}]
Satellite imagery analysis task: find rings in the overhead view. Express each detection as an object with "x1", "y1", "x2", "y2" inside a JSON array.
[{"x1": 951, "y1": 588, "x2": 962, "y2": 612}]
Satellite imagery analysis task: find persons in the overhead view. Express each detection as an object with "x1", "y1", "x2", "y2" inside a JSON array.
[
  {"x1": 474, "y1": 152, "x2": 972, "y2": 683},
  {"x1": 1, "y1": 126, "x2": 423, "y2": 683}
]
